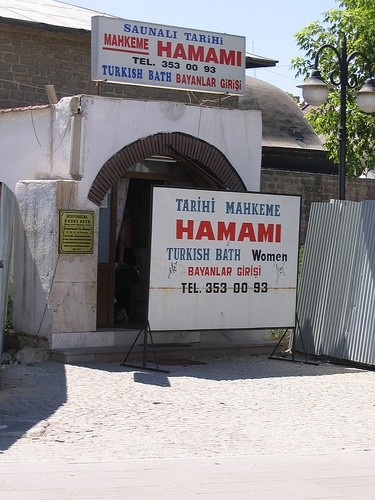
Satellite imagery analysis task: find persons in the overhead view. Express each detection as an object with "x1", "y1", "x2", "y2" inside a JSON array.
[{"x1": 115, "y1": 205, "x2": 137, "y2": 324}]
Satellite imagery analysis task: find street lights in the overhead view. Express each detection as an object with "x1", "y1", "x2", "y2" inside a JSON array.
[{"x1": 297, "y1": 29, "x2": 374, "y2": 201}]
